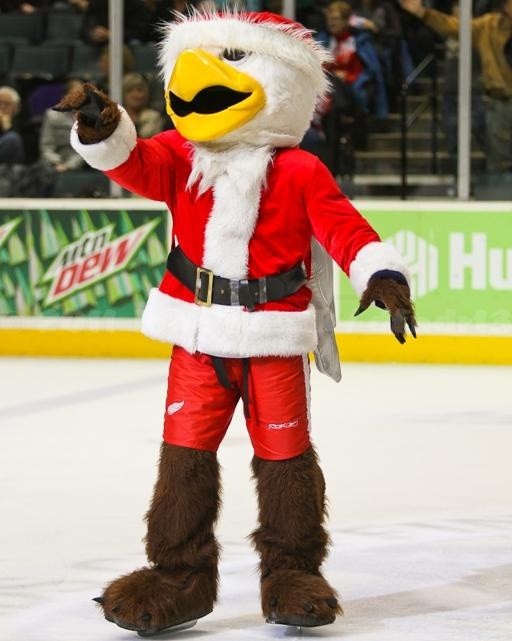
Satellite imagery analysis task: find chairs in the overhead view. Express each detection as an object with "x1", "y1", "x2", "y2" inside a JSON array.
[{"x1": 0, "y1": 0, "x2": 372, "y2": 199}]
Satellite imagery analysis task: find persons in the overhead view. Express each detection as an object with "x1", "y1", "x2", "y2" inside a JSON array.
[{"x1": 0, "y1": 1, "x2": 509, "y2": 205}]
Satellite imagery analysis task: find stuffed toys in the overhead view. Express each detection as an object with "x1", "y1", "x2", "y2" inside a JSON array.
[{"x1": 49, "y1": 1, "x2": 421, "y2": 635}]
